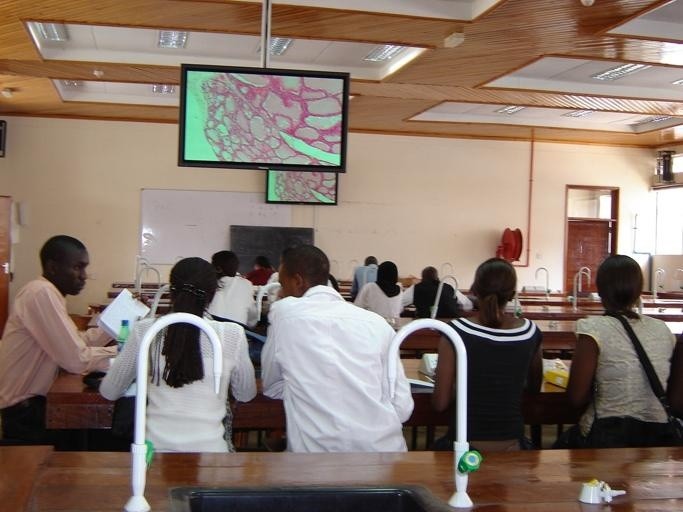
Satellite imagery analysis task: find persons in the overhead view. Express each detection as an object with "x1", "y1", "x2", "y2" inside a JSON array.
[
  {"x1": 257, "y1": 244, "x2": 415, "y2": 452},
  {"x1": 98, "y1": 256, "x2": 257, "y2": 452},
  {"x1": 402, "y1": 266, "x2": 473, "y2": 319},
  {"x1": 263, "y1": 237, "x2": 337, "y2": 296},
  {"x1": 0, "y1": 234, "x2": 153, "y2": 445},
  {"x1": 245, "y1": 256, "x2": 275, "y2": 285},
  {"x1": 552, "y1": 257, "x2": 675, "y2": 448},
  {"x1": 205, "y1": 252, "x2": 262, "y2": 332},
  {"x1": 352, "y1": 263, "x2": 403, "y2": 324},
  {"x1": 431, "y1": 258, "x2": 544, "y2": 451},
  {"x1": 665, "y1": 332, "x2": 682, "y2": 409},
  {"x1": 348, "y1": 253, "x2": 379, "y2": 298}
]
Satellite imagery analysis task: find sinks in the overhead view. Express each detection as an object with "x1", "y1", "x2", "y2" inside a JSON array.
[
  {"x1": 668, "y1": 290, "x2": 683, "y2": 293},
  {"x1": 168, "y1": 486, "x2": 456, "y2": 512},
  {"x1": 584, "y1": 309, "x2": 604, "y2": 312}
]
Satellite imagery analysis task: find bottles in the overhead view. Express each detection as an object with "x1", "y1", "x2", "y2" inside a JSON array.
[
  {"x1": 578, "y1": 482, "x2": 603, "y2": 504},
  {"x1": 117, "y1": 319, "x2": 131, "y2": 354}
]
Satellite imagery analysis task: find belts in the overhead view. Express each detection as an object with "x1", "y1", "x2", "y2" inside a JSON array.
[{"x1": 7, "y1": 398, "x2": 41, "y2": 415}]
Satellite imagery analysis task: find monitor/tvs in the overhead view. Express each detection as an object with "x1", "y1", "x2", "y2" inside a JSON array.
[
  {"x1": 177, "y1": 63, "x2": 350, "y2": 172},
  {"x1": 265, "y1": 170, "x2": 339, "y2": 206}
]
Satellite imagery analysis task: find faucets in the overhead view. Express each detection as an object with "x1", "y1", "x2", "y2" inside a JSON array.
[
  {"x1": 135, "y1": 256, "x2": 161, "y2": 293},
  {"x1": 149, "y1": 284, "x2": 171, "y2": 315},
  {"x1": 125, "y1": 313, "x2": 221, "y2": 512},
  {"x1": 579, "y1": 267, "x2": 590, "y2": 292},
  {"x1": 440, "y1": 263, "x2": 454, "y2": 276},
  {"x1": 672, "y1": 269, "x2": 683, "y2": 290},
  {"x1": 573, "y1": 272, "x2": 591, "y2": 308},
  {"x1": 256, "y1": 283, "x2": 281, "y2": 321},
  {"x1": 430, "y1": 275, "x2": 459, "y2": 318},
  {"x1": 388, "y1": 318, "x2": 482, "y2": 508},
  {"x1": 653, "y1": 269, "x2": 666, "y2": 297},
  {"x1": 536, "y1": 267, "x2": 550, "y2": 291}
]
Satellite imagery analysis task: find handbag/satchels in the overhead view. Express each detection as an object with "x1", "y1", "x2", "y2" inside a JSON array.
[
  {"x1": 111, "y1": 396, "x2": 135, "y2": 446},
  {"x1": 663, "y1": 400, "x2": 683, "y2": 446}
]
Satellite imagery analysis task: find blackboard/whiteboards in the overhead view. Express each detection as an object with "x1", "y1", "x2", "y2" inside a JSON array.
[{"x1": 140, "y1": 188, "x2": 292, "y2": 266}]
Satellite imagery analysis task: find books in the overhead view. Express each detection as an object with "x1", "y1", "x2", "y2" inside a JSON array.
[
  {"x1": 96, "y1": 288, "x2": 150, "y2": 341},
  {"x1": 420, "y1": 350, "x2": 440, "y2": 376}
]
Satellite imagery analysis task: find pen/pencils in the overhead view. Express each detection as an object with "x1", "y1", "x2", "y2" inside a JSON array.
[{"x1": 425, "y1": 375, "x2": 435, "y2": 383}]
